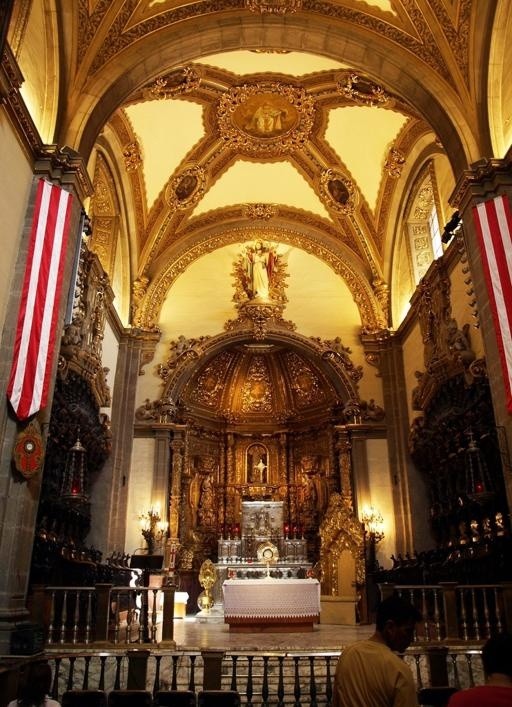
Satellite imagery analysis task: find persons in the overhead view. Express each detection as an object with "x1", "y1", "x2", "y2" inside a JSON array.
[
  {"x1": 251, "y1": 98, "x2": 290, "y2": 137},
  {"x1": 7, "y1": 659, "x2": 62, "y2": 707},
  {"x1": 447, "y1": 628, "x2": 512, "y2": 707},
  {"x1": 253, "y1": 508, "x2": 269, "y2": 532},
  {"x1": 332, "y1": 595, "x2": 426, "y2": 707},
  {"x1": 243, "y1": 239, "x2": 276, "y2": 303}
]
